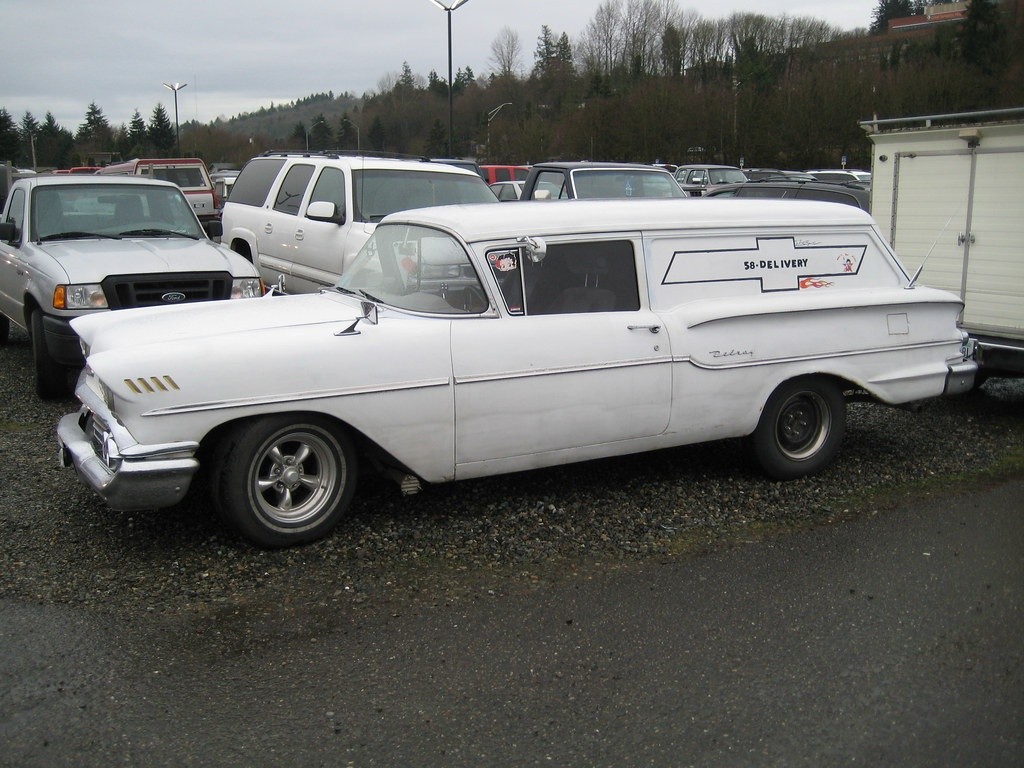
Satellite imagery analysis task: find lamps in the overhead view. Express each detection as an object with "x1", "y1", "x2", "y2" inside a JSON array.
[{"x1": 959, "y1": 130, "x2": 981, "y2": 141}]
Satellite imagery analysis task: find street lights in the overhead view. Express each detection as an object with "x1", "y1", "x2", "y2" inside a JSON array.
[
  {"x1": 429, "y1": 0, "x2": 469, "y2": 160},
  {"x1": 163, "y1": 83, "x2": 187, "y2": 157},
  {"x1": 306, "y1": 119, "x2": 360, "y2": 153},
  {"x1": 487, "y1": 103, "x2": 512, "y2": 162}
]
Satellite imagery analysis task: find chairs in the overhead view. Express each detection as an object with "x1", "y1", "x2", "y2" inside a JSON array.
[
  {"x1": 111, "y1": 195, "x2": 144, "y2": 226},
  {"x1": 502, "y1": 246, "x2": 548, "y2": 310},
  {"x1": 543, "y1": 242, "x2": 621, "y2": 316},
  {"x1": 36, "y1": 190, "x2": 67, "y2": 234}
]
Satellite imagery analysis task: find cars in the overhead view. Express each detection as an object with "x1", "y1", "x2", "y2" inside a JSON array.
[
  {"x1": 56, "y1": 198, "x2": 977, "y2": 548},
  {"x1": 0, "y1": 149, "x2": 872, "y2": 401}
]
